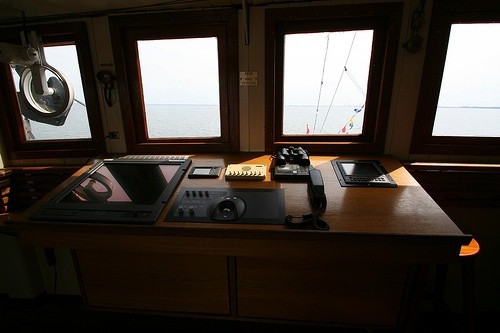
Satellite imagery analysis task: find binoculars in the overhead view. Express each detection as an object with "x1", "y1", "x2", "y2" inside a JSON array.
[{"x1": 275, "y1": 145, "x2": 310, "y2": 167}]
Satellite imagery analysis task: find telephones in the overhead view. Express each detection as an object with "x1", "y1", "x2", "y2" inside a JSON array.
[{"x1": 308, "y1": 169, "x2": 327, "y2": 201}]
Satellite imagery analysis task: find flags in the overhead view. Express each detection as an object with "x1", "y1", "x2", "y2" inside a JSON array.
[
  {"x1": 342, "y1": 126, "x2": 346, "y2": 132},
  {"x1": 354, "y1": 108, "x2": 361, "y2": 113},
  {"x1": 350, "y1": 117, "x2": 354, "y2": 129},
  {"x1": 306, "y1": 126, "x2": 309, "y2": 134}
]
